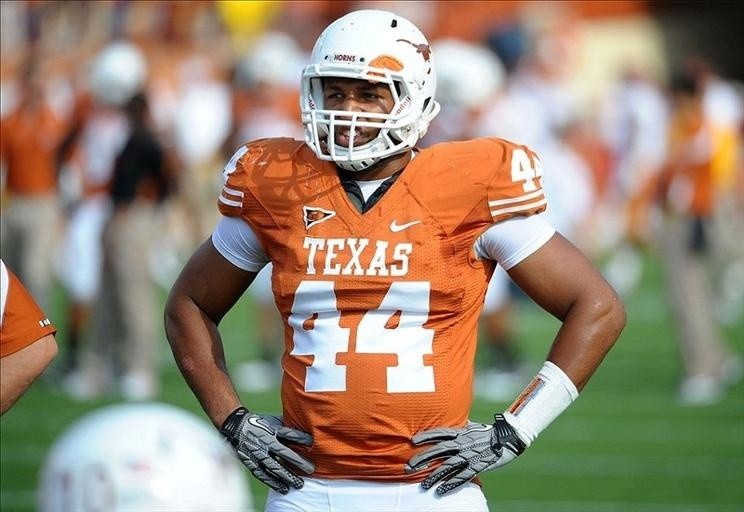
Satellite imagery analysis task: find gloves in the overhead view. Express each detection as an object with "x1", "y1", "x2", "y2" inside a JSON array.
[
  {"x1": 406, "y1": 414, "x2": 526, "y2": 495},
  {"x1": 220, "y1": 407, "x2": 315, "y2": 495}
]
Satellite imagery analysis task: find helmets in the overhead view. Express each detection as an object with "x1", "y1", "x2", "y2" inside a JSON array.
[{"x1": 298, "y1": 9, "x2": 441, "y2": 172}]
